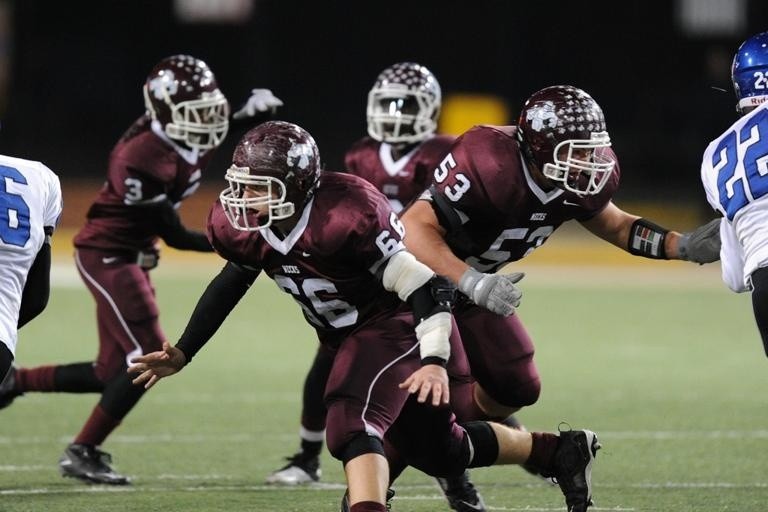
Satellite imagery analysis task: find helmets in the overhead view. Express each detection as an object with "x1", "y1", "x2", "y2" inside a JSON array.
[
  {"x1": 516, "y1": 85, "x2": 616, "y2": 198},
  {"x1": 732, "y1": 32, "x2": 768, "y2": 112},
  {"x1": 143, "y1": 55, "x2": 321, "y2": 232},
  {"x1": 365, "y1": 62, "x2": 442, "y2": 149}
]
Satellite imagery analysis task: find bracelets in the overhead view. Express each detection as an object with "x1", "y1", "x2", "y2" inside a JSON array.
[{"x1": 625, "y1": 214, "x2": 670, "y2": 262}]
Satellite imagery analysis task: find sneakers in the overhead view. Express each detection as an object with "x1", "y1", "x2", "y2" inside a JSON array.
[
  {"x1": 437, "y1": 472, "x2": 487, "y2": 512},
  {"x1": 268, "y1": 451, "x2": 322, "y2": 485},
  {"x1": 59, "y1": 443, "x2": 127, "y2": 485},
  {"x1": 550, "y1": 430, "x2": 601, "y2": 512}
]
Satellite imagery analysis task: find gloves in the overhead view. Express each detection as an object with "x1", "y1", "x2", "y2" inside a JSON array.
[
  {"x1": 457, "y1": 268, "x2": 524, "y2": 317},
  {"x1": 679, "y1": 218, "x2": 720, "y2": 264}
]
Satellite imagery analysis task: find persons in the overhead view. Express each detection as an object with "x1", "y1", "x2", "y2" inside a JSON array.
[
  {"x1": 0, "y1": 153, "x2": 63, "y2": 379},
  {"x1": 0, "y1": 55, "x2": 229, "y2": 484},
  {"x1": 265, "y1": 62, "x2": 487, "y2": 512},
  {"x1": 341, "y1": 86, "x2": 722, "y2": 512},
  {"x1": 701, "y1": 33, "x2": 767, "y2": 357},
  {"x1": 126, "y1": 119, "x2": 602, "y2": 512}
]
